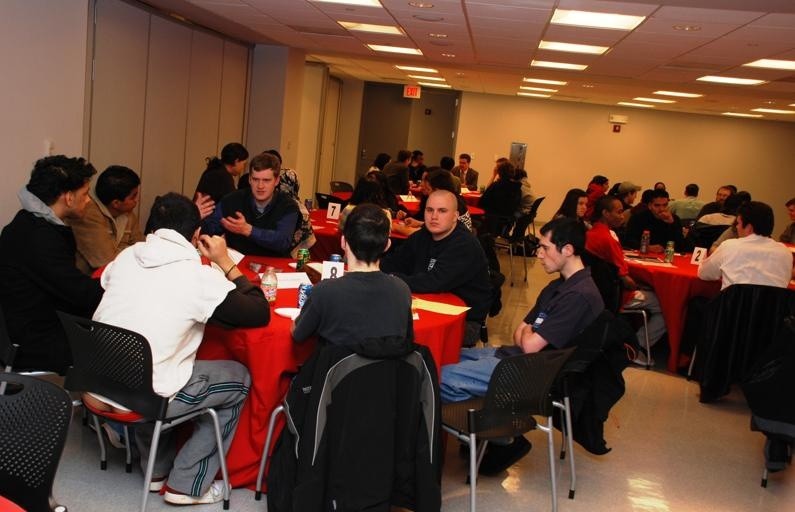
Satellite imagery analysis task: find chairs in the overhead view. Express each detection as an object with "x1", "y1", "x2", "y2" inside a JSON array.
[
  {"x1": 582, "y1": 247, "x2": 653, "y2": 371},
  {"x1": 57, "y1": 309, "x2": 233, "y2": 512},
  {"x1": 254, "y1": 344, "x2": 442, "y2": 512},
  {"x1": 494, "y1": 212, "x2": 536, "y2": 286},
  {"x1": 526, "y1": 196, "x2": 546, "y2": 242},
  {"x1": 550, "y1": 249, "x2": 624, "y2": 503},
  {"x1": 0, "y1": 369, "x2": 75, "y2": 512},
  {"x1": 686, "y1": 283, "x2": 795, "y2": 407},
  {"x1": 441, "y1": 347, "x2": 578, "y2": 512},
  {"x1": 314, "y1": 192, "x2": 345, "y2": 210},
  {"x1": 477, "y1": 204, "x2": 517, "y2": 286},
  {"x1": 329, "y1": 180, "x2": 355, "y2": 193}
]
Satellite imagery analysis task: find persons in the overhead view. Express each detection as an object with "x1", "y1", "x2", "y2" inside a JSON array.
[
  {"x1": 378, "y1": 189, "x2": 493, "y2": 349},
  {"x1": 195, "y1": 153, "x2": 300, "y2": 260},
  {"x1": 439, "y1": 157, "x2": 461, "y2": 194},
  {"x1": 1, "y1": 156, "x2": 103, "y2": 377},
  {"x1": 681, "y1": 192, "x2": 746, "y2": 252},
  {"x1": 451, "y1": 153, "x2": 479, "y2": 191},
  {"x1": 485, "y1": 157, "x2": 511, "y2": 188},
  {"x1": 583, "y1": 196, "x2": 668, "y2": 367},
  {"x1": 408, "y1": 150, "x2": 424, "y2": 189},
  {"x1": 477, "y1": 162, "x2": 523, "y2": 228},
  {"x1": 74, "y1": 165, "x2": 147, "y2": 279},
  {"x1": 778, "y1": 198, "x2": 795, "y2": 246},
  {"x1": 512, "y1": 168, "x2": 534, "y2": 218},
  {"x1": 81, "y1": 192, "x2": 272, "y2": 507},
  {"x1": 617, "y1": 188, "x2": 654, "y2": 230},
  {"x1": 291, "y1": 203, "x2": 416, "y2": 374},
  {"x1": 697, "y1": 201, "x2": 794, "y2": 290},
  {"x1": 552, "y1": 188, "x2": 592, "y2": 232},
  {"x1": 438, "y1": 216, "x2": 605, "y2": 475},
  {"x1": 585, "y1": 175, "x2": 609, "y2": 222},
  {"x1": 262, "y1": 150, "x2": 316, "y2": 257},
  {"x1": 708, "y1": 203, "x2": 746, "y2": 256},
  {"x1": 727, "y1": 184, "x2": 752, "y2": 204},
  {"x1": 409, "y1": 164, "x2": 432, "y2": 204},
  {"x1": 608, "y1": 179, "x2": 666, "y2": 201},
  {"x1": 374, "y1": 150, "x2": 414, "y2": 211},
  {"x1": 350, "y1": 152, "x2": 392, "y2": 203},
  {"x1": 625, "y1": 190, "x2": 683, "y2": 254},
  {"x1": 696, "y1": 186, "x2": 735, "y2": 223},
  {"x1": 667, "y1": 183, "x2": 707, "y2": 224},
  {"x1": 425, "y1": 169, "x2": 473, "y2": 233},
  {"x1": 192, "y1": 142, "x2": 251, "y2": 211}
]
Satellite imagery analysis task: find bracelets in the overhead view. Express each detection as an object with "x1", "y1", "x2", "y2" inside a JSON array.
[{"x1": 225, "y1": 263, "x2": 237, "y2": 278}]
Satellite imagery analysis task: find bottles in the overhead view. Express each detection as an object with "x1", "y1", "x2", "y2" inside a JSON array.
[
  {"x1": 298, "y1": 284, "x2": 312, "y2": 308},
  {"x1": 641, "y1": 229, "x2": 650, "y2": 257},
  {"x1": 664, "y1": 240, "x2": 674, "y2": 263},
  {"x1": 331, "y1": 254, "x2": 342, "y2": 262},
  {"x1": 305, "y1": 199, "x2": 313, "y2": 213},
  {"x1": 261, "y1": 266, "x2": 277, "y2": 302},
  {"x1": 297, "y1": 249, "x2": 311, "y2": 269}
]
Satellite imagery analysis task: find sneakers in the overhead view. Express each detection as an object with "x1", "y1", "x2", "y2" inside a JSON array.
[
  {"x1": 102, "y1": 417, "x2": 127, "y2": 457},
  {"x1": 163, "y1": 477, "x2": 232, "y2": 506},
  {"x1": 633, "y1": 350, "x2": 656, "y2": 366},
  {"x1": 473, "y1": 434, "x2": 532, "y2": 477},
  {"x1": 147, "y1": 477, "x2": 169, "y2": 492}
]
